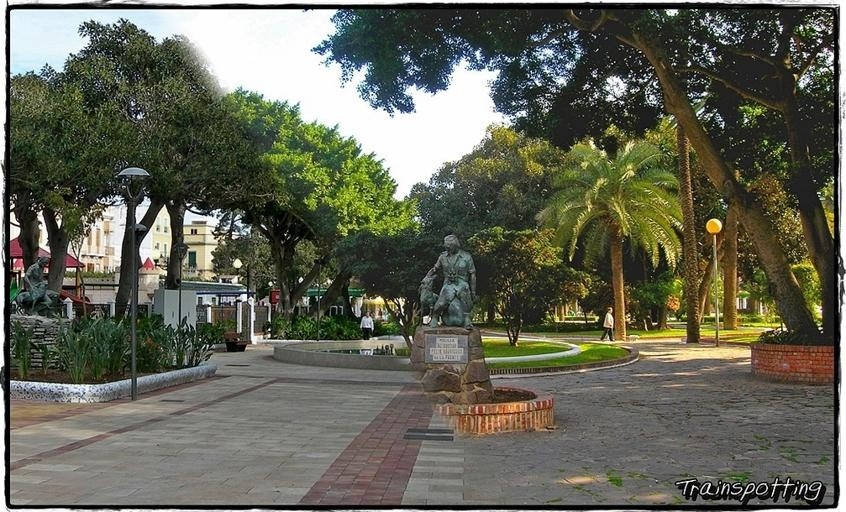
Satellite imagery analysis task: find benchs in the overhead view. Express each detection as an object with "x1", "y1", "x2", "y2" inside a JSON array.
[{"x1": 224, "y1": 332, "x2": 248, "y2": 352}]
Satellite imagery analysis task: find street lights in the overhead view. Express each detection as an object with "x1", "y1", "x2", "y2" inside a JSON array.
[
  {"x1": 118, "y1": 167, "x2": 150, "y2": 401},
  {"x1": 232, "y1": 257, "x2": 243, "y2": 284},
  {"x1": 174, "y1": 239, "x2": 190, "y2": 324},
  {"x1": 706, "y1": 218, "x2": 722, "y2": 347}
]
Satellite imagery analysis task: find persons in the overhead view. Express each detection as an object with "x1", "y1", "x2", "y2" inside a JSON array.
[
  {"x1": 600, "y1": 307, "x2": 615, "y2": 341},
  {"x1": 24, "y1": 256, "x2": 52, "y2": 311},
  {"x1": 568, "y1": 306, "x2": 575, "y2": 317},
  {"x1": 426, "y1": 234, "x2": 477, "y2": 330},
  {"x1": 360, "y1": 311, "x2": 374, "y2": 340}
]
